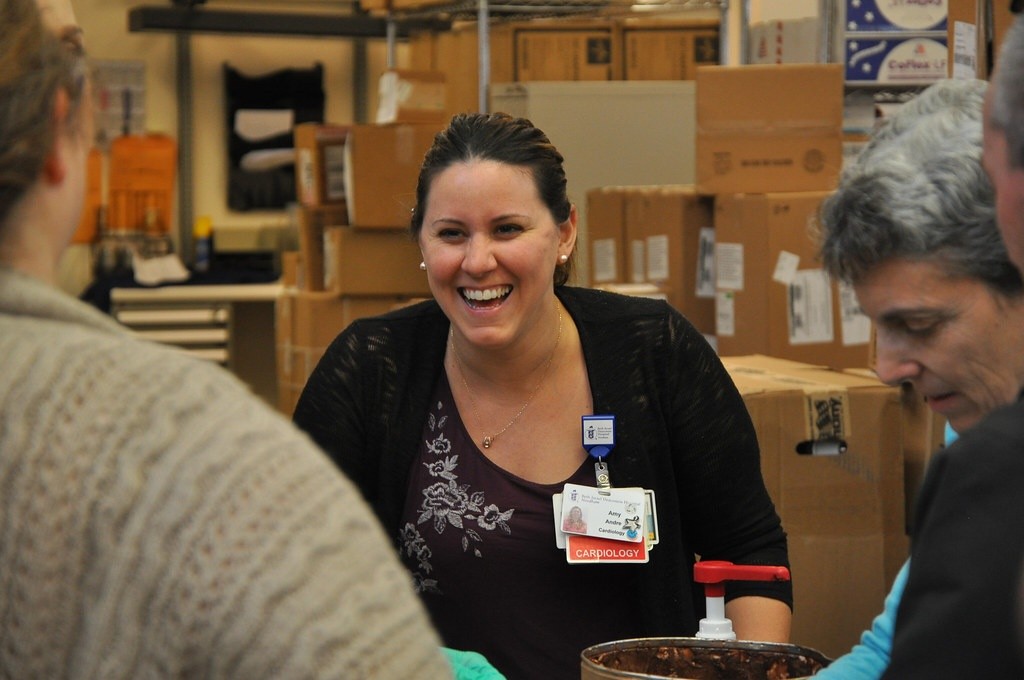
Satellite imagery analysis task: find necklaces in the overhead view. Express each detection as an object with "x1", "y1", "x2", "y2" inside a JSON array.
[{"x1": 451, "y1": 301, "x2": 562, "y2": 448}]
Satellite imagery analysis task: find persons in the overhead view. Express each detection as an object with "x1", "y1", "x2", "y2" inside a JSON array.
[
  {"x1": 808, "y1": 77, "x2": 1024, "y2": 680},
  {"x1": 0, "y1": 0, "x2": 452, "y2": 680},
  {"x1": 563, "y1": 506, "x2": 588, "y2": 534},
  {"x1": 870, "y1": 14, "x2": 1024, "y2": 680},
  {"x1": 290, "y1": 111, "x2": 794, "y2": 680}
]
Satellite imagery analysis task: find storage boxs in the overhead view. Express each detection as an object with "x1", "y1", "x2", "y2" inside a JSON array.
[{"x1": 274, "y1": 0, "x2": 1024, "y2": 660}]
